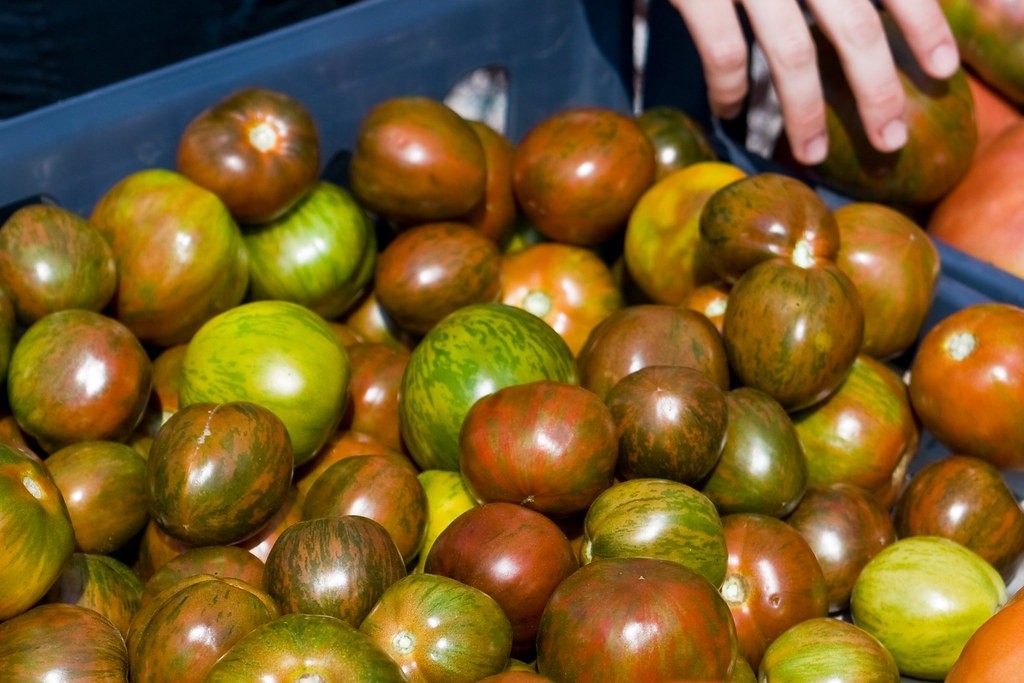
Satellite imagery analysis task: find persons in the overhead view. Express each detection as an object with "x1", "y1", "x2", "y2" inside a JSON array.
[{"x1": 0, "y1": 1, "x2": 962, "y2": 169}]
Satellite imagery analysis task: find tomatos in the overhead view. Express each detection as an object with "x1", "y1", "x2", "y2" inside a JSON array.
[{"x1": 0, "y1": 0, "x2": 1024, "y2": 683}]
